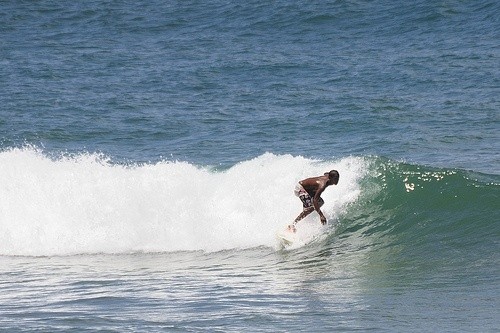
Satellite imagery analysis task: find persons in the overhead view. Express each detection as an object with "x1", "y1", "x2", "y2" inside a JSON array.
[{"x1": 289, "y1": 170, "x2": 339, "y2": 232}]
